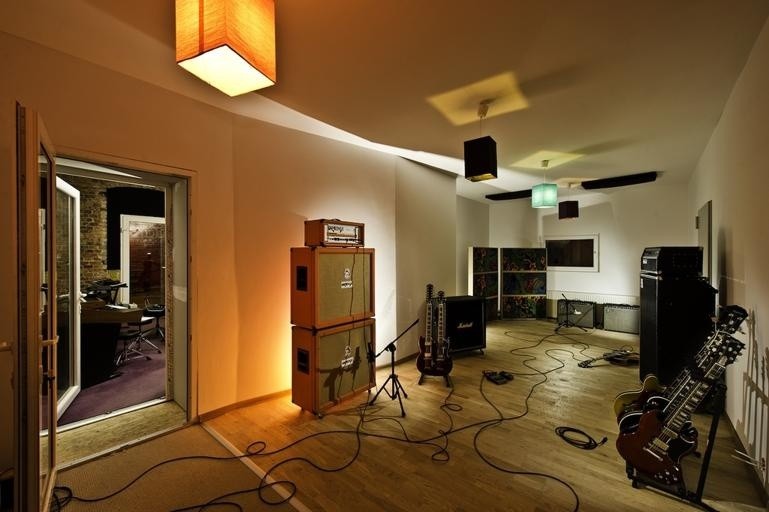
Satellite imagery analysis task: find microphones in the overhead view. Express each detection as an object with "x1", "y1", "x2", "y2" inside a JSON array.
[{"x1": 368, "y1": 343, "x2": 374, "y2": 369}]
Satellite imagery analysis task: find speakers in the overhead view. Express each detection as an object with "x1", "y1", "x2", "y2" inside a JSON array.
[
  {"x1": 603, "y1": 304, "x2": 640, "y2": 334},
  {"x1": 431, "y1": 295, "x2": 486, "y2": 355},
  {"x1": 289, "y1": 247, "x2": 375, "y2": 328},
  {"x1": 639, "y1": 273, "x2": 715, "y2": 386},
  {"x1": 291, "y1": 317, "x2": 377, "y2": 420},
  {"x1": 556, "y1": 299, "x2": 595, "y2": 328}
]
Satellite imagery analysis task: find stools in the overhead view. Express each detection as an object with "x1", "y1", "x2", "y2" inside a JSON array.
[{"x1": 114, "y1": 305, "x2": 166, "y2": 367}]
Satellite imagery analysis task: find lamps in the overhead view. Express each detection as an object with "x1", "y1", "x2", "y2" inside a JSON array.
[
  {"x1": 531, "y1": 159, "x2": 579, "y2": 220},
  {"x1": 175, "y1": 0, "x2": 279, "y2": 99},
  {"x1": 463, "y1": 100, "x2": 498, "y2": 181}
]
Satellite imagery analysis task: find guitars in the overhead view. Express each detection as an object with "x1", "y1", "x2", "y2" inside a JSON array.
[{"x1": 614, "y1": 302, "x2": 747, "y2": 486}]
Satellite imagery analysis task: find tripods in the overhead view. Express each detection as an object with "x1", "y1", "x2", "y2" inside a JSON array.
[
  {"x1": 554, "y1": 293, "x2": 587, "y2": 332},
  {"x1": 369, "y1": 318, "x2": 419, "y2": 417}
]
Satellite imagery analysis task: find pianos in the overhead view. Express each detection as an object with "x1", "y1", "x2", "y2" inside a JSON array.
[{"x1": 54, "y1": 289, "x2": 143, "y2": 326}]
